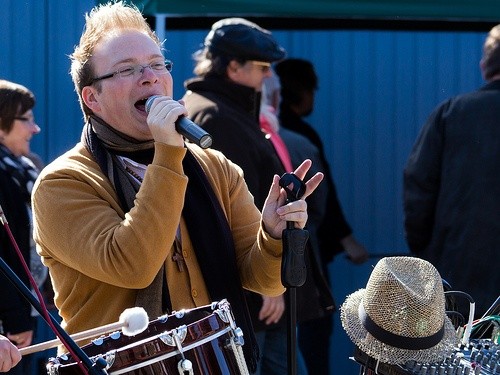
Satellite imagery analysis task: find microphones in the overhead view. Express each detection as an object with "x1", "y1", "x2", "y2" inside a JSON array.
[{"x1": 145, "y1": 95, "x2": 213, "y2": 149}]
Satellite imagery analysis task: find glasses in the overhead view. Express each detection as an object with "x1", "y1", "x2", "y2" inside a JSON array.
[
  {"x1": 246, "y1": 60, "x2": 271, "y2": 73},
  {"x1": 88, "y1": 60, "x2": 173, "y2": 86},
  {"x1": 14, "y1": 114, "x2": 34, "y2": 125}
]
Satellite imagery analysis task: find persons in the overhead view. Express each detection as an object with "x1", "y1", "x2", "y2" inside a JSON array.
[
  {"x1": 31, "y1": 0, "x2": 324, "y2": 356},
  {"x1": 404, "y1": 24, "x2": 500, "y2": 341},
  {"x1": 0, "y1": 19, "x2": 371, "y2": 375}
]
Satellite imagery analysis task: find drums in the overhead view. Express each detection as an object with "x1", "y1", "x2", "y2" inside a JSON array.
[{"x1": 46, "y1": 300, "x2": 251, "y2": 375}]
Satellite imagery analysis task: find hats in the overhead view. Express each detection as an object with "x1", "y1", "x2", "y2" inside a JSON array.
[
  {"x1": 204, "y1": 18, "x2": 286, "y2": 63},
  {"x1": 339, "y1": 256, "x2": 457, "y2": 363}
]
